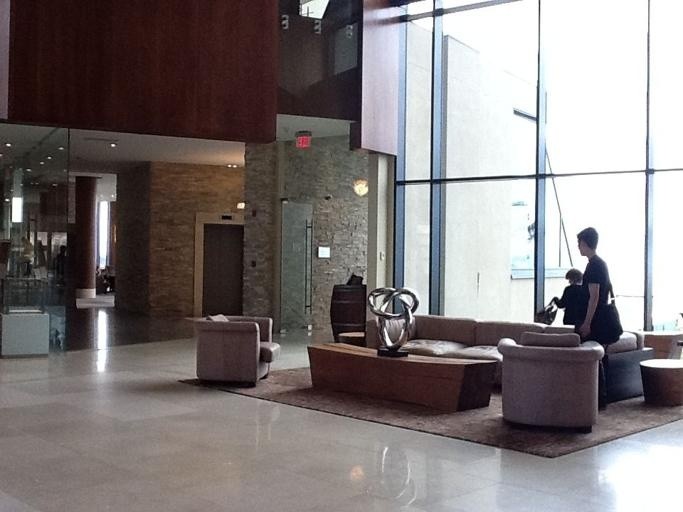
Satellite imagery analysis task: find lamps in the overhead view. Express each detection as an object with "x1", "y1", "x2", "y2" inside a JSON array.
[{"x1": 352, "y1": 178, "x2": 369, "y2": 197}]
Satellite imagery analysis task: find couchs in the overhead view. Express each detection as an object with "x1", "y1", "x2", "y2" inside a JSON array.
[{"x1": 365, "y1": 313, "x2": 654, "y2": 405}]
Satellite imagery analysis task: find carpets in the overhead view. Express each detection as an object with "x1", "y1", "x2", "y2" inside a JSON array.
[{"x1": 177, "y1": 363, "x2": 683, "y2": 459}]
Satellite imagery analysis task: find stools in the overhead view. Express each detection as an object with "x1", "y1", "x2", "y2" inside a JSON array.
[
  {"x1": 641, "y1": 330, "x2": 681, "y2": 360},
  {"x1": 639, "y1": 358, "x2": 683, "y2": 406},
  {"x1": 336, "y1": 332, "x2": 365, "y2": 347}
]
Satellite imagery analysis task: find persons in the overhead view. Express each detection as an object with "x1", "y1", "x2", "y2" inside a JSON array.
[
  {"x1": 576, "y1": 225, "x2": 612, "y2": 412},
  {"x1": 554, "y1": 268, "x2": 584, "y2": 325},
  {"x1": 56, "y1": 245, "x2": 68, "y2": 258},
  {"x1": 20, "y1": 235, "x2": 34, "y2": 277}
]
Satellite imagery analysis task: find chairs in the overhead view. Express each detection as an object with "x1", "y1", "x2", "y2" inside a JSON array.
[
  {"x1": 497, "y1": 332, "x2": 605, "y2": 435},
  {"x1": 194, "y1": 313, "x2": 281, "y2": 388}
]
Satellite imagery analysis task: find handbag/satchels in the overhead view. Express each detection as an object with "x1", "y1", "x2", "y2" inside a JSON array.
[
  {"x1": 590, "y1": 304, "x2": 623, "y2": 344},
  {"x1": 535, "y1": 297, "x2": 557, "y2": 324}
]
